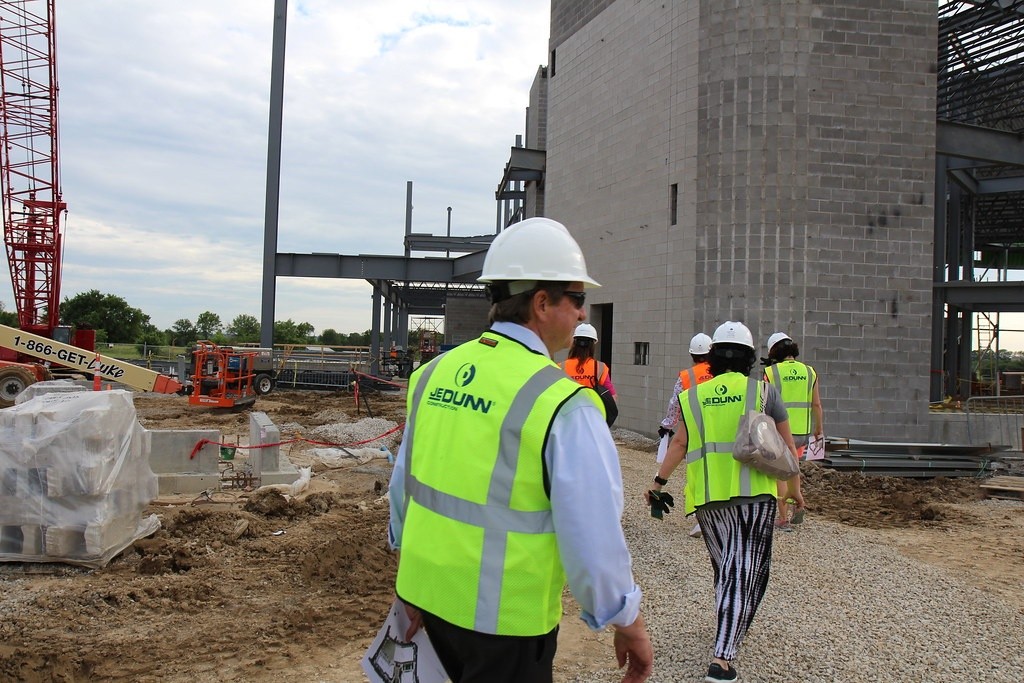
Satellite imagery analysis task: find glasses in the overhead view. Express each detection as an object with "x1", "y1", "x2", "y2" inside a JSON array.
[{"x1": 529, "y1": 288, "x2": 586, "y2": 310}]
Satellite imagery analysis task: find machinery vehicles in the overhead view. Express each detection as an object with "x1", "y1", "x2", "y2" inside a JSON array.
[{"x1": 2, "y1": 0, "x2": 260, "y2": 415}]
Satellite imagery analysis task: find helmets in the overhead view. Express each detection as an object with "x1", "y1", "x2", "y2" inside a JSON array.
[
  {"x1": 709, "y1": 321, "x2": 754, "y2": 350},
  {"x1": 573, "y1": 323, "x2": 598, "y2": 344},
  {"x1": 475, "y1": 217, "x2": 602, "y2": 288},
  {"x1": 767, "y1": 332, "x2": 793, "y2": 356},
  {"x1": 689, "y1": 332, "x2": 712, "y2": 354}
]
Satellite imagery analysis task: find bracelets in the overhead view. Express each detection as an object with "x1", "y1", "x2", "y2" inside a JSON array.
[{"x1": 654, "y1": 476, "x2": 667, "y2": 485}]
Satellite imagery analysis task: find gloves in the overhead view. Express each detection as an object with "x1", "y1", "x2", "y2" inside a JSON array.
[
  {"x1": 759, "y1": 357, "x2": 778, "y2": 366},
  {"x1": 657, "y1": 426, "x2": 674, "y2": 438},
  {"x1": 648, "y1": 490, "x2": 674, "y2": 520}
]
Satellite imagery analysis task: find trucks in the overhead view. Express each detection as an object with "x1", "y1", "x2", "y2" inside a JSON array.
[{"x1": 189, "y1": 344, "x2": 275, "y2": 395}]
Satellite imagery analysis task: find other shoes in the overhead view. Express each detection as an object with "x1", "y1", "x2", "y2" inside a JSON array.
[
  {"x1": 704, "y1": 662, "x2": 737, "y2": 683},
  {"x1": 689, "y1": 523, "x2": 702, "y2": 538},
  {"x1": 789, "y1": 505, "x2": 805, "y2": 524},
  {"x1": 774, "y1": 519, "x2": 791, "y2": 531}
]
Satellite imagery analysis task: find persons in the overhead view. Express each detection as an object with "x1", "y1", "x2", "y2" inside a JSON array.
[
  {"x1": 557, "y1": 323, "x2": 617, "y2": 401},
  {"x1": 646, "y1": 321, "x2": 804, "y2": 683},
  {"x1": 659, "y1": 332, "x2": 713, "y2": 538},
  {"x1": 388, "y1": 218, "x2": 653, "y2": 683},
  {"x1": 760, "y1": 332, "x2": 823, "y2": 528}
]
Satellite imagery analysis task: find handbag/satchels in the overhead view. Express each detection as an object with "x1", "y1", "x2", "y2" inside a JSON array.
[
  {"x1": 590, "y1": 360, "x2": 618, "y2": 428},
  {"x1": 733, "y1": 378, "x2": 800, "y2": 481}
]
